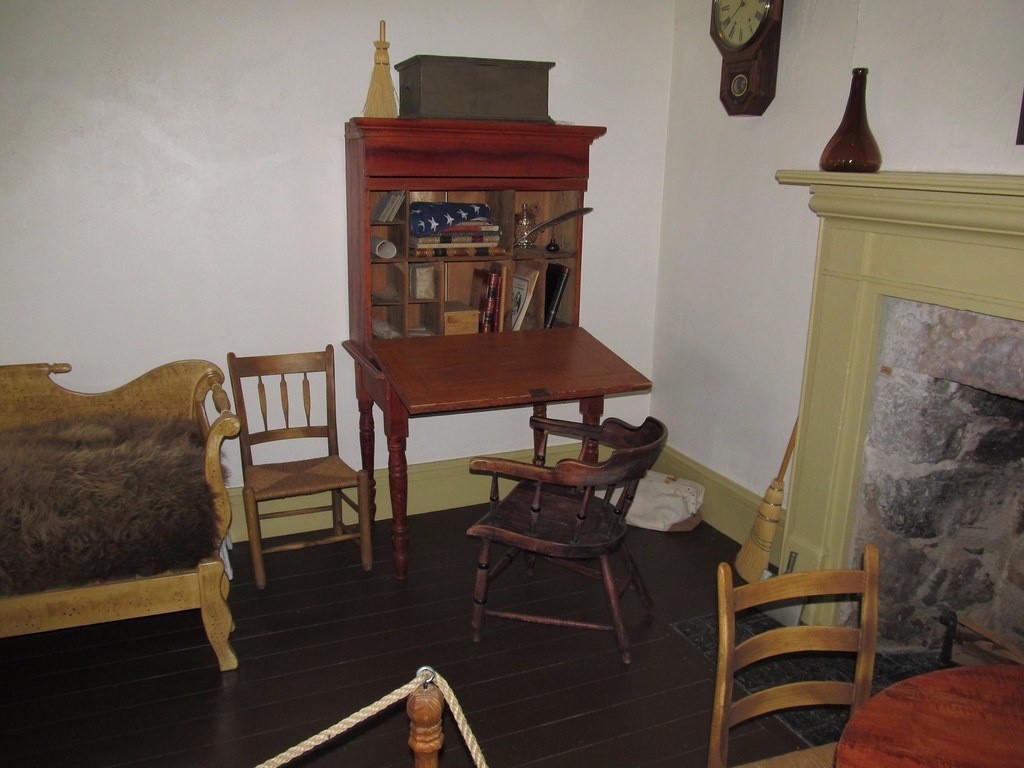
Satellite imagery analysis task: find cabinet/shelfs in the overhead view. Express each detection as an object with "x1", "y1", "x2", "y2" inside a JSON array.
[{"x1": 343, "y1": 117, "x2": 653, "y2": 579}]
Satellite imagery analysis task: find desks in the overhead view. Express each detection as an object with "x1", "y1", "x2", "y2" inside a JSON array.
[{"x1": 833, "y1": 665, "x2": 1024, "y2": 768}]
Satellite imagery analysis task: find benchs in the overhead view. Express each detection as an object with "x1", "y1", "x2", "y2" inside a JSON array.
[{"x1": 0, "y1": 359, "x2": 242, "y2": 672}]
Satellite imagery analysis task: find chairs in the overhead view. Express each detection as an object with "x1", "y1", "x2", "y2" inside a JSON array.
[
  {"x1": 227, "y1": 344, "x2": 372, "y2": 590},
  {"x1": 707, "y1": 543, "x2": 879, "y2": 768},
  {"x1": 466, "y1": 416, "x2": 669, "y2": 664}
]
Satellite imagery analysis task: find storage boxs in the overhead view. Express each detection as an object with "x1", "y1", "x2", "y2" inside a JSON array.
[
  {"x1": 444, "y1": 301, "x2": 480, "y2": 336},
  {"x1": 394, "y1": 55, "x2": 556, "y2": 124}
]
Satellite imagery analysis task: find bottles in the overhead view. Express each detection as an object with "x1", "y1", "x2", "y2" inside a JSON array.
[
  {"x1": 515, "y1": 204, "x2": 538, "y2": 248},
  {"x1": 818, "y1": 67, "x2": 882, "y2": 174}
]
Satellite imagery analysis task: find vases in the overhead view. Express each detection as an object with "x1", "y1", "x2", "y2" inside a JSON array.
[{"x1": 818, "y1": 67, "x2": 881, "y2": 172}]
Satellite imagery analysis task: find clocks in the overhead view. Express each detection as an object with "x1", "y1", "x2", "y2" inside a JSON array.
[{"x1": 708, "y1": 0, "x2": 784, "y2": 116}]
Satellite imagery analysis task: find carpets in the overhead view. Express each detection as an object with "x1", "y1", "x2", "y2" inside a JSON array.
[{"x1": 668, "y1": 605, "x2": 962, "y2": 749}]
